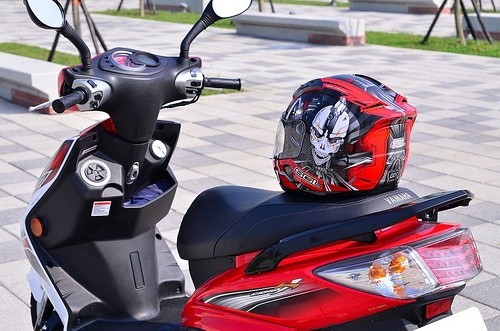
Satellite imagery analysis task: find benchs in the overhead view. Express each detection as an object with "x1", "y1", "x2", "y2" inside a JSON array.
[
  {"x1": 462, "y1": 11, "x2": 500, "y2": 41},
  {"x1": 0, "y1": 51, "x2": 75, "y2": 115},
  {"x1": 347, "y1": 0, "x2": 455, "y2": 14},
  {"x1": 231, "y1": 10, "x2": 366, "y2": 46}
]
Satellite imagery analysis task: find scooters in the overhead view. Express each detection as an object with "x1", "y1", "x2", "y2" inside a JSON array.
[{"x1": 16, "y1": 0, "x2": 494, "y2": 330}]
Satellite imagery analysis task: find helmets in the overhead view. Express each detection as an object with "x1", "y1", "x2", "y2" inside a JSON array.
[{"x1": 273, "y1": 73, "x2": 418, "y2": 196}]
